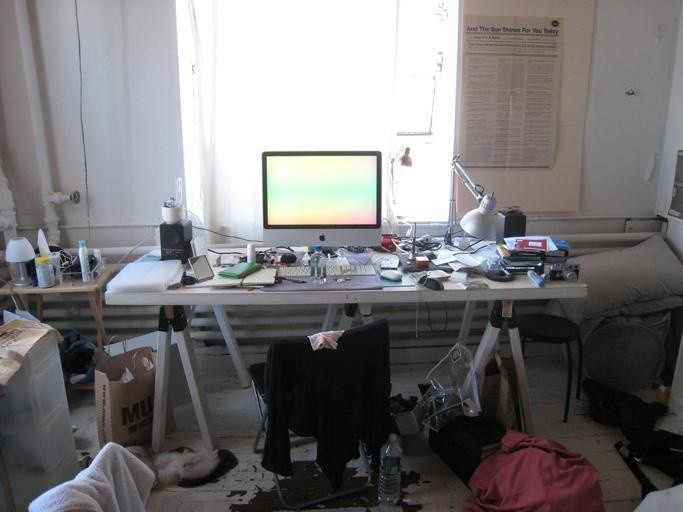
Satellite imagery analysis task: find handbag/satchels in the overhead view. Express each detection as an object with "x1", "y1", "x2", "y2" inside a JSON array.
[{"x1": 94, "y1": 334, "x2": 176, "y2": 448}]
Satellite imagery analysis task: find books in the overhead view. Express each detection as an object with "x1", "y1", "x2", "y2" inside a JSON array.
[{"x1": 497, "y1": 236, "x2": 559, "y2": 273}]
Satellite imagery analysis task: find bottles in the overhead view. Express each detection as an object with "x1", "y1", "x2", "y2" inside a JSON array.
[
  {"x1": 77, "y1": 240, "x2": 94, "y2": 282},
  {"x1": 377, "y1": 433, "x2": 403, "y2": 506},
  {"x1": 34, "y1": 258, "x2": 57, "y2": 288},
  {"x1": 308, "y1": 245, "x2": 324, "y2": 286}
]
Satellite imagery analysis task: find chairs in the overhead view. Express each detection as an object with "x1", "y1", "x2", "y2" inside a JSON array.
[{"x1": 249, "y1": 319, "x2": 392, "y2": 508}]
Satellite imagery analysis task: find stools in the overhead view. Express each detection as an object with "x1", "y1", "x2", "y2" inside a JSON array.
[{"x1": 514, "y1": 313, "x2": 583, "y2": 429}]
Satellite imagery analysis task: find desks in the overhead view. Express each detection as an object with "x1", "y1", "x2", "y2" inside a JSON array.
[
  {"x1": 105, "y1": 245, "x2": 590, "y2": 459},
  {"x1": 1, "y1": 269, "x2": 116, "y2": 397}
]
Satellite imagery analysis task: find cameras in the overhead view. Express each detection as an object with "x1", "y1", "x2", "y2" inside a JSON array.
[{"x1": 550, "y1": 263, "x2": 579, "y2": 282}]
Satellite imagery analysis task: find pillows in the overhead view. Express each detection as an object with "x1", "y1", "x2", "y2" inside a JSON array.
[
  {"x1": 550, "y1": 234, "x2": 683, "y2": 326},
  {"x1": 443, "y1": 156, "x2": 497, "y2": 245}
]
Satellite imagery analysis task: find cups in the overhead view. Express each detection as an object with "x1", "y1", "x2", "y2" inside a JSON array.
[{"x1": 162, "y1": 205, "x2": 183, "y2": 224}]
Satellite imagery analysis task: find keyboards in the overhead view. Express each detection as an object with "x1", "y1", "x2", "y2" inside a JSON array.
[{"x1": 278, "y1": 264, "x2": 377, "y2": 278}]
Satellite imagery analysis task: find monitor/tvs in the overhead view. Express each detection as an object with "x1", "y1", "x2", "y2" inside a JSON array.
[{"x1": 261, "y1": 151, "x2": 382, "y2": 266}]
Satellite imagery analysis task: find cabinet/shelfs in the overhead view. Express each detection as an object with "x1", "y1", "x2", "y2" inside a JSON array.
[{"x1": 0, "y1": 328, "x2": 81, "y2": 508}]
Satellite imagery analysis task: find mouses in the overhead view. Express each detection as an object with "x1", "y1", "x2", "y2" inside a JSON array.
[{"x1": 381, "y1": 270, "x2": 403, "y2": 281}]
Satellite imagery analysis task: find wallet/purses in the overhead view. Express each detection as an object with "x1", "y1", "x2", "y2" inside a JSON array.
[{"x1": 218, "y1": 262, "x2": 262, "y2": 279}]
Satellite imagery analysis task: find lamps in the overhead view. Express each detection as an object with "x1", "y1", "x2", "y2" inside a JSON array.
[{"x1": 5, "y1": 238, "x2": 36, "y2": 287}]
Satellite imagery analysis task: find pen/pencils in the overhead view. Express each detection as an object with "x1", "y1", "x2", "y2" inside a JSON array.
[{"x1": 277, "y1": 277, "x2": 306, "y2": 283}]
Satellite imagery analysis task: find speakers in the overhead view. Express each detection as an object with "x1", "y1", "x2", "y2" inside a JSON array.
[
  {"x1": 496, "y1": 208, "x2": 527, "y2": 245},
  {"x1": 159, "y1": 219, "x2": 192, "y2": 265}
]
